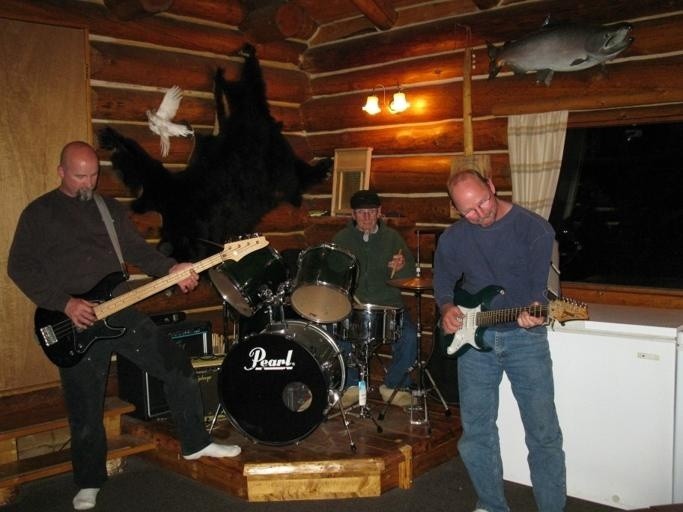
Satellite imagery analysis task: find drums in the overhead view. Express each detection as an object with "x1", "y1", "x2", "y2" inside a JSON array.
[
  {"x1": 330, "y1": 303, "x2": 406, "y2": 345},
  {"x1": 217, "y1": 319, "x2": 346, "y2": 446},
  {"x1": 288, "y1": 240, "x2": 359, "y2": 324},
  {"x1": 208, "y1": 244, "x2": 287, "y2": 317}
]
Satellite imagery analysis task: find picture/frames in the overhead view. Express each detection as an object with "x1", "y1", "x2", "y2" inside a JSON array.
[{"x1": 330, "y1": 145, "x2": 374, "y2": 217}]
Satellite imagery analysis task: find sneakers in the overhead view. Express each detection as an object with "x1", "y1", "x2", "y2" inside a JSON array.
[
  {"x1": 379, "y1": 384, "x2": 418, "y2": 407},
  {"x1": 335, "y1": 387, "x2": 359, "y2": 409}
]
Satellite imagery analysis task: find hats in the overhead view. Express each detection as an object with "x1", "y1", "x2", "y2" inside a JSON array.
[{"x1": 349, "y1": 189, "x2": 382, "y2": 209}]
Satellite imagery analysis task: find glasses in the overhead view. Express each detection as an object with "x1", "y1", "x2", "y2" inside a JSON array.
[
  {"x1": 352, "y1": 209, "x2": 380, "y2": 216},
  {"x1": 463, "y1": 194, "x2": 493, "y2": 219}
]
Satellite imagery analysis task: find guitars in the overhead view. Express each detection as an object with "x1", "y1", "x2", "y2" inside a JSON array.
[
  {"x1": 33, "y1": 231, "x2": 270, "y2": 368},
  {"x1": 433, "y1": 285, "x2": 590, "y2": 359}
]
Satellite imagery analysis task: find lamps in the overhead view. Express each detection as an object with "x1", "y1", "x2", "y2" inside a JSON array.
[{"x1": 360, "y1": 81, "x2": 411, "y2": 115}]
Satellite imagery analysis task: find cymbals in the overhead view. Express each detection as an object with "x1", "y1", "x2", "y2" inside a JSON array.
[{"x1": 388, "y1": 277, "x2": 434, "y2": 292}]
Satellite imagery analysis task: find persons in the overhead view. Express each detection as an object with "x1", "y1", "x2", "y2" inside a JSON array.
[
  {"x1": 329, "y1": 189, "x2": 418, "y2": 410},
  {"x1": 431, "y1": 169, "x2": 567, "y2": 512},
  {"x1": 7, "y1": 141, "x2": 242, "y2": 511}
]
